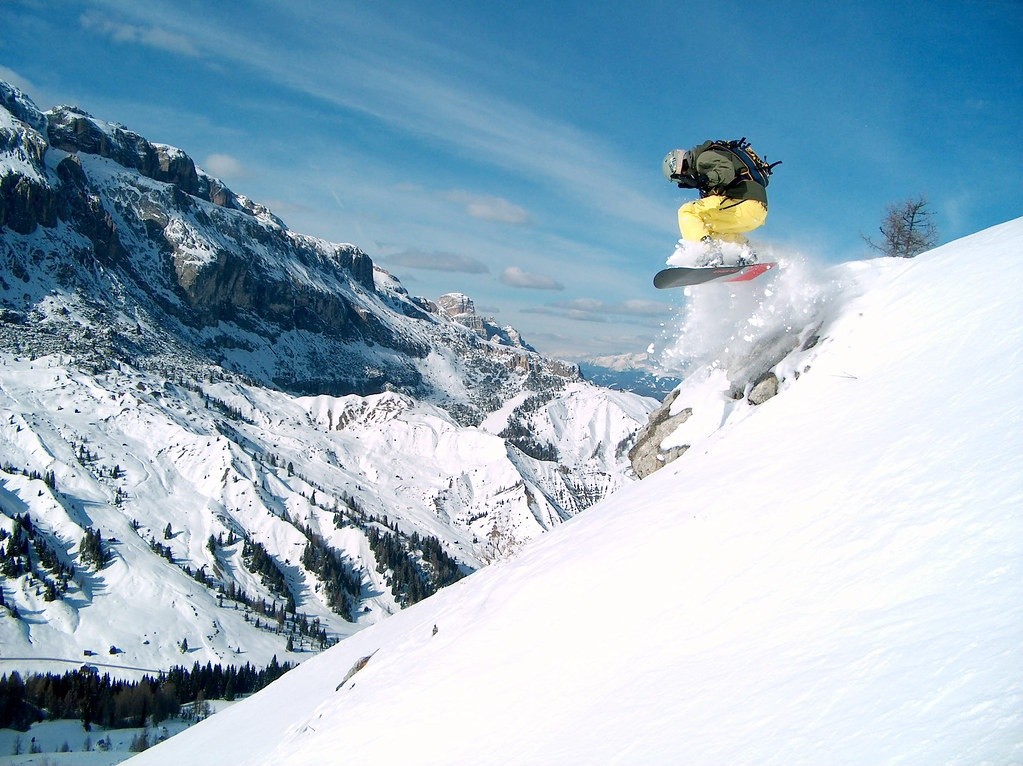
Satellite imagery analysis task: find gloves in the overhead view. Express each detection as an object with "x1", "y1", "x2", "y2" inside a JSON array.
[{"x1": 670, "y1": 171, "x2": 709, "y2": 189}]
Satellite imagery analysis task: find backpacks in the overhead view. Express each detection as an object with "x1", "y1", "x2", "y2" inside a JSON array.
[{"x1": 704, "y1": 137, "x2": 773, "y2": 189}]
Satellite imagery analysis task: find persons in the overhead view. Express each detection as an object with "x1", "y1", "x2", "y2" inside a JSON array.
[
  {"x1": 287, "y1": 462, "x2": 295, "y2": 477},
  {"x1": 165, "y1": 522, "x2": 173, "y2": 539},
  {"x1": 663, "y1": 140, "x2": 769, "y2": 266}
]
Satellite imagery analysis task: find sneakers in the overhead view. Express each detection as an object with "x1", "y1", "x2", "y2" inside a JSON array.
[
  {"x1": 728, "y1": 250, "x2": 759, "y2": 267},
  {"x1": 693, "y1": 245, "x2": 724, "y2": 267}
]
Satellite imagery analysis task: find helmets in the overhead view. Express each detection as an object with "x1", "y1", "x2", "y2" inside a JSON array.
[{"x1": 662, "y1": 148, "x2": 687, "y2": 184}]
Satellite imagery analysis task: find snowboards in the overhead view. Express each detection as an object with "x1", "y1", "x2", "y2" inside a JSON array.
[{"x1": 653, "y1": 261, "x2": 778, "y2": 289}]
